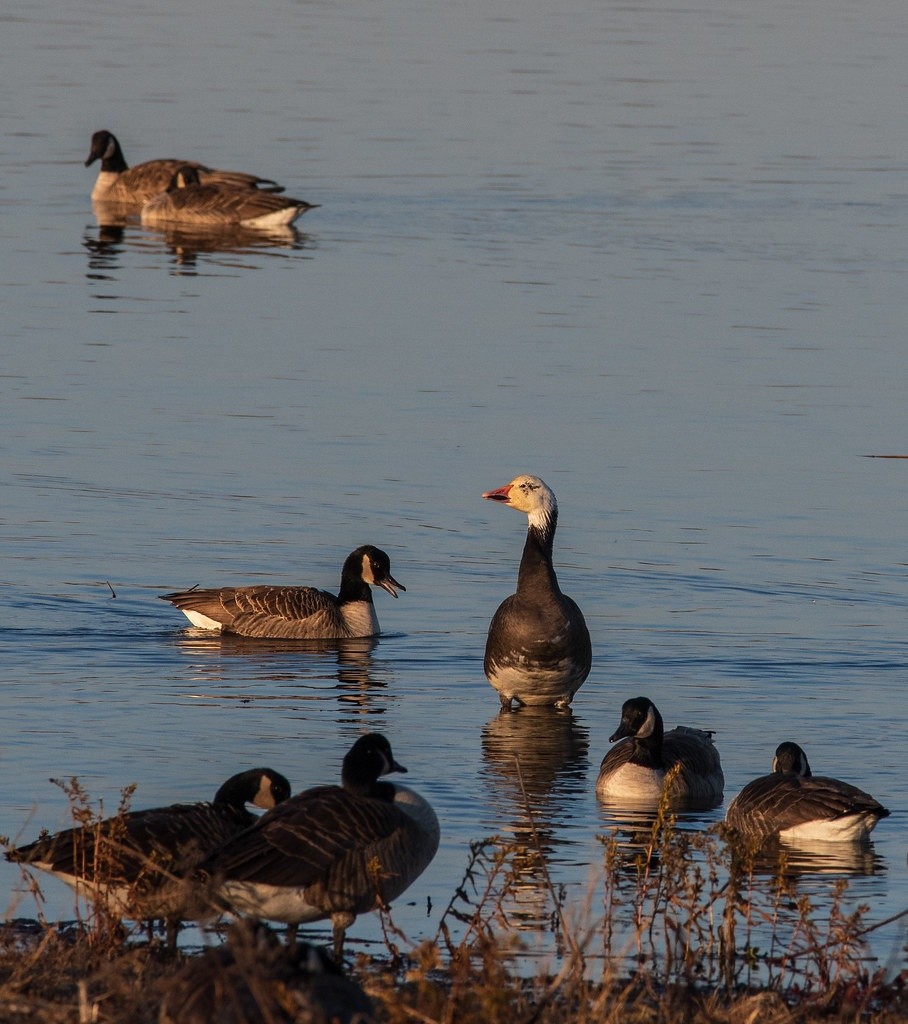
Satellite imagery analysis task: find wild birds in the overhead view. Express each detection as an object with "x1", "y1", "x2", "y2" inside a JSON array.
[
  {"x1": 478, "y1": 473, "x2": 592, "y2": 715},
  {"x1": 184, "y1": 734, "x2": 442, "y2": 979},
  {"x1": 3, "y1": 768, "x2": 293, "y2": 954},
  {"x1": 596, "y1": 696, "x2": 725, "y2": 810},
  {"x1": 723, "y1": 741, "x2": 891, "y2": 843},
  {"x1": 158, "y1": 545, "x2": 408, "y2": 640},
  {"x1": 82, "y1": 130, "x2": 286, "y2": 203},
  {"x1": 140, "y1": 165, "x2": 323, "y2": 225}
]
